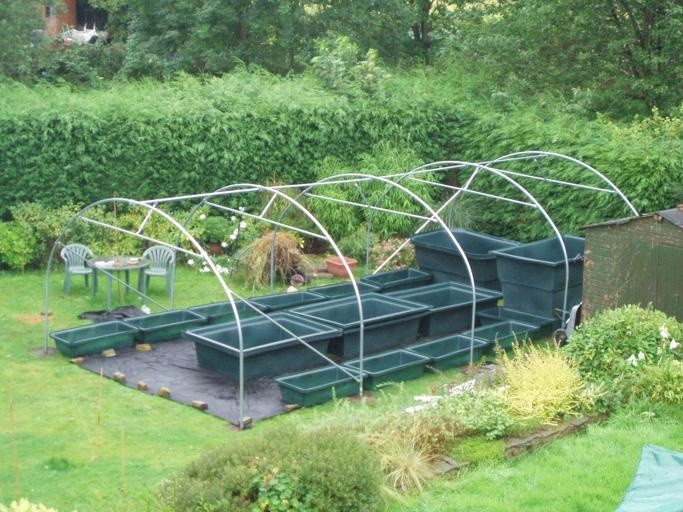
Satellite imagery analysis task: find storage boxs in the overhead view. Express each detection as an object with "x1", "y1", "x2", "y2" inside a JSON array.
[
  {"x1": 462, "y1": 320, "x2": 540, "y2": 345},
  {"x1": 288, "y1": 292, "x2": 429, "y2": 359},
  {"x1": 48, "y1": 319, "x2": 139, "y2": 359},
  {"x1": 123, "y1": 309, "x2": 208, "y2": 344},
  {"x1": 361, "y1": 267, "x2": 432, "y2": 294},
  {"x1": 187, "y1": 299, "x2": 268, "y2": 326},
  {"x1": 477, "y1": 305, "x2": 556, "y2": 338},
  {"x1": 249, "y1": 290, "x2": 327, "y2": 312},
  {"x1": 343, "y1": 349, "x2": 432, "y2": 391},
  {"x1": 275, "y1": 365, "x2": 368, "y2": 408},
  {"x1": 184, "y1": 311, "x2": 343, "y2": 382},
  {"x1": 383, "y1": 281, "x2": 503, "y2": 338},
  {"x1": 405, "y1": 334, "x2": 489, "y2": 370},
  {"x1": 410, "y1": 227, "x2": 524, "y2": 282},
  {"x1": 488, "y1": 234, "x2": 585, "y2": 319},
  {"x1": 307, "y1": 281, "x2": 381, "y2": 301}
]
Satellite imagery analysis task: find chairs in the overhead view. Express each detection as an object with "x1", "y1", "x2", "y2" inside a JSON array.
[
  {"x1": 138, "y1": 245, "x2": 174, "y2": 295},
  {"x1": 60, "y1": 243, "x2": 96, "y2": 293}
]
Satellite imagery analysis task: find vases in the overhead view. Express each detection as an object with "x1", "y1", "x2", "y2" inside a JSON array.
[{"x1": 324, "y1": 255, "x2": 358, "y2": 277}]
